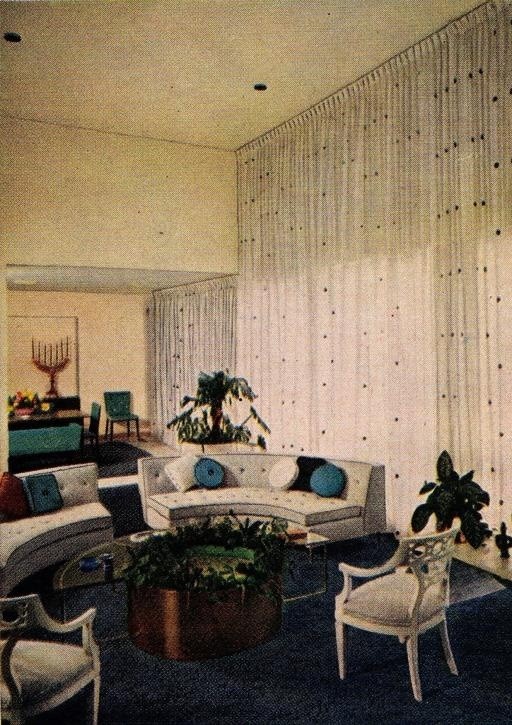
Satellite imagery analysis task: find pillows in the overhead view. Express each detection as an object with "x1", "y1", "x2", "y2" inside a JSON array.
[
  {"x1": 20, "y1": 473, "x2": 65, "y2": 515},
  {"x1": 270, "y1": 454, "x2": 346, "y2": 498},
  {"x1": 0, "y1": 471, "x2": 33, "y2": 524},
  {"x1": 165, "y1": 453, "x2": 209, "y2": 492},
  {"x1": 194, "y1": 457, "x2": 227, "y2": 488}
]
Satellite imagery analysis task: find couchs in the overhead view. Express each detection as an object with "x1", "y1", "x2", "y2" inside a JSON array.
[
  {"x1": 0, "y1": 461, "x2": 113, "y2": 601},
  {"x1": 136, "y1": 452, "x2": 390, "y2": 548}
]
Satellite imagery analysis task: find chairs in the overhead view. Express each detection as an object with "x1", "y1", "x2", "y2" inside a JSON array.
[
  {"x1": 331, "y1": 513, "x2": 469, "y2": 704},
  {"x1": 7, "y1": 390, "x2": 141, "y2": 458},
  {"x1": 0, "y1": 592, "x2": 106, "y2": 724}
]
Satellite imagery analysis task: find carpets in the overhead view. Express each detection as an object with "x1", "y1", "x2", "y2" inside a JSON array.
[
  {"x1": 1, "y1": 481, "x2": 511, "y2": 723},
  {"x1": 8, "y1": 439, "x2": 157, "y2": 478}
]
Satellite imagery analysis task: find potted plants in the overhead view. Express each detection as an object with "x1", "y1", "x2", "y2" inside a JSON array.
[
  {"x1": 164, "y1": 362, "x2": 271, "y2": 454},
  {"x1": 124, "y1": 511, "x2": 311, "y2": 663}
]
